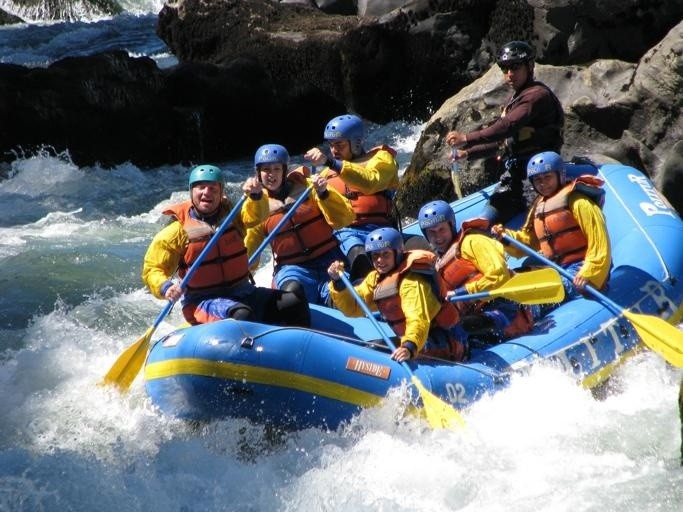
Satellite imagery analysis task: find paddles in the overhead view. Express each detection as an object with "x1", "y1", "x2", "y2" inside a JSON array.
[
  {"x1": 99, "y1": 191, "x2": 250, "y2": 397},
  {"x1": 442, "y1": 268, "x2": 566, "y2": 305},
  {"x1": 336, "y1": 261, "x2": 465, "y2": 432},
  {"x1": 449, "y1": 144, "x2": 464, "y2": 200},
  {"x1": 492, "y1": 227, "x2": 683, "y2": 368}
]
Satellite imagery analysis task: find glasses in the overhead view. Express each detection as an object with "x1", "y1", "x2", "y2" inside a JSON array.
[{"x1": 500, "y1": 63, "x2": 526, "y2": 72}]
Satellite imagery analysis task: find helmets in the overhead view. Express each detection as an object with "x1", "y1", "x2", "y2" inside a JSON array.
[
  {"x1": 418, "y1": 201, "x2": 458, "y2": 242},
  {"x1": 187, "y1": 164, "x2": 226, "y2": 187},
  {"x1": 322, "y1": 115, "x2": 366, "y2": 154},
  {"x1": 527, "y1": 151, "x2": 567, "y2": 186},
  {"x1": 499, "y1": 41, "x2": 535, "y2": 65},
  {"x1": 253, "y1": 142, "x2": 292, "y2": 166},
  {"x1": 365, "y1": 227, "x2": 404, "y2": 266}
]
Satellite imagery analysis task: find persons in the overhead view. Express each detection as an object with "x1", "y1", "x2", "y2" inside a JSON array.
[
  {"x1": 142, "y1": 165, "x2": 310, "y2": 328},
  {"x1": 491, "y1": 152, "x2": 612, "y2": 317},
  {"x1": 446, "y1": 41, "x2": 565, "y2": 229},
  {"x1": 327, "y1": 228, "x2": 471, "y2": 364},
  {"x1": 418, "y1": 201, "x2": 535, "y2": 346},
  {"x1": 304, "y1": 114, "x2": 430, "y2": 283},
  {"x1": 243, "y1": 144, "x2": 355, "y2": 309}
]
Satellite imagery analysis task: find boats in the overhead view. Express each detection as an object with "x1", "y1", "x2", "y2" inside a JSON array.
[{"x1": 143, "y1": 163, "x2": 682, "y2": 430}]
